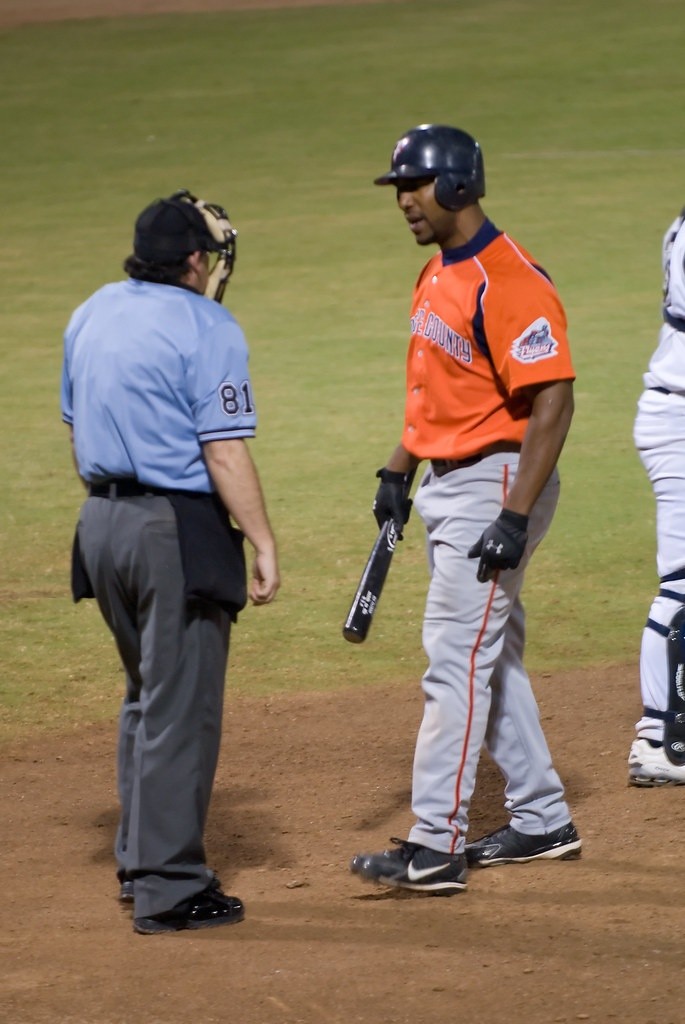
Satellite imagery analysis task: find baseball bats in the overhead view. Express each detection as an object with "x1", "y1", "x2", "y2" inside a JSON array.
[{"x1": 341, "y1": 463, "x2": 421, "y2": 645}]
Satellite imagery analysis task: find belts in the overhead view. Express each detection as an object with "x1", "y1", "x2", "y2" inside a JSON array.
[
  {"x1": 652, "y1": 387, "x2": 671, "y2": 394},
  {"x1": 431, "y1": 442, "x2": 521, "y2": 477},
  {"x1": 91, "y1": 478, "x2": 168, "y2": 497}
]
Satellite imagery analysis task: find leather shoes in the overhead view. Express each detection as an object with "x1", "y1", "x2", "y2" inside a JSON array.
[
  {"x1": 119, "y1": 874, "x2": 134, "y2": 899},
  {"x1": 133, "y1": 876, "x2": 244, "y2": 934}
]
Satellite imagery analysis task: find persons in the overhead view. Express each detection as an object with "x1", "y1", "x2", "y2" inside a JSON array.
[
  {"x1": 58, "y1": 189, "x2": 282, "y2": 937},
  {"x1": 627, "y1": 207, "x2": 685, "y2": 789},
  {"x1": 350, "y1": 124, "x2": 584, "y2": 891}
]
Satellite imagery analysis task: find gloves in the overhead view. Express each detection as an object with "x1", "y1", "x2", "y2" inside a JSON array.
[
  {"x1": 468, "y1": 508, "x2": 528, "y2": 583},
  {"x1": 373, "y1": 467, "x2": 412, "y2": 540}
]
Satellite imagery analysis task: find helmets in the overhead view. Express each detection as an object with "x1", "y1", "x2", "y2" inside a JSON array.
[{"x1": 374, "y1": 124, "x2": 485, "y2": 212}]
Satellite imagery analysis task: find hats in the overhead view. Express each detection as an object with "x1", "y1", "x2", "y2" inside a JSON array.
[{"x1": 134, "y1": 198, "x2": 218, "y2": 252}]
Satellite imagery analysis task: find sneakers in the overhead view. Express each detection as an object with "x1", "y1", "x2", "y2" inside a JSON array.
[
  {"x1": 628, "y1": 738, "x2": 685, "y2": 785},
  {"x1": 465, "y1": 823, "x2": 582, "y2": 867},
  {"x1": 353, "y1": 838, "x2": 468, "y2": 891}
]
